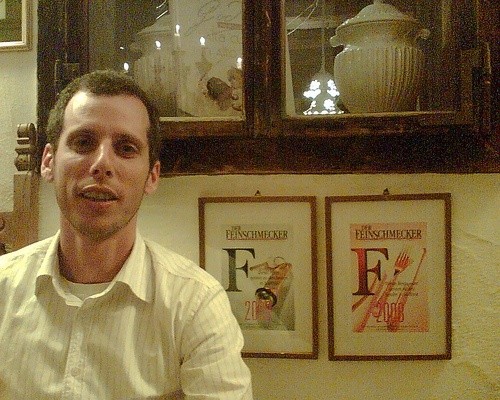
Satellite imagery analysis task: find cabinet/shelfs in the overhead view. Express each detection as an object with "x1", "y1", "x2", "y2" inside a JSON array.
[{"x1": 37, "y1": 0, "x2": 500, "y2": 178}]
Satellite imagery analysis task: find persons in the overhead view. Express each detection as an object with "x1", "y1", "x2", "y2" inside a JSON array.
[{"x1": 2, "y1": 68, "x2": 256, "y2": 399}]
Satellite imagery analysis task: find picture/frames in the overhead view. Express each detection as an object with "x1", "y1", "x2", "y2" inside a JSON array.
[
  {"x1": 324, "y1": 192, "x2": 452, "y2": 362},
  {"x1": 199, "y1": 194, "x2": 319, "y2": 360},
  {"x1": 0, "y1": 0, "x2": 34, "y2": 53}
]
